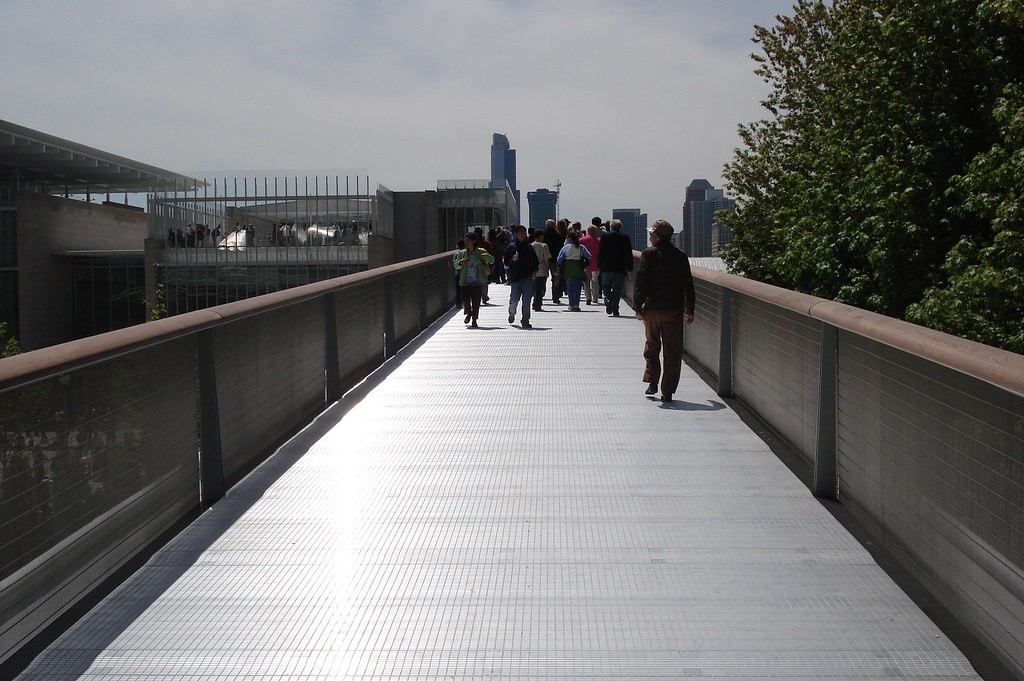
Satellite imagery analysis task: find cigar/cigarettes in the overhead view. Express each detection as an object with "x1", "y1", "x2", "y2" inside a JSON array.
[{"x1": 516, "y1": 251, "x2": 518, "y2": 255}]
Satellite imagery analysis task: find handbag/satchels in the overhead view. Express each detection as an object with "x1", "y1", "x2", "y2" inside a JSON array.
[{"x1": 480, "y1": 248, "x2": 491, "y2": 276}]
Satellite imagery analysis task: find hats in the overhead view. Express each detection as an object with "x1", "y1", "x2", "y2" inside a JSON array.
[
  {"x1": 561, "y1": 219, "x2": 571, "y2": 223},
  {"x1": 646, "y1": 220, "x2": 674, "y2": 238}
]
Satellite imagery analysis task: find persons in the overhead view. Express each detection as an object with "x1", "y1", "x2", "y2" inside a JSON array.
[
  {"x1": 453, "y1": 239, "x2": 465, "y2": 309},
  {"x1": 211, "y1": 224, "x2": 221, "y2": 248},
  {"x1": 454, "y1": 232, "x2": 497, "y2": 328},
  {"x1": 232, "y1": 221, "x2": 361, "y2": 246},
  {"x1": 473, "y1": 226, "x2": 495, "y2": 304},
  {"x1": 488, "y1": 217, "x2": 611, "y2": 312},
  {"x1": 167, "y1": 223, "x2": 211, "y2": 247},
  {"x1": 503, "y1": 224, "x2": 540, "y2": 328},
  {"x1": 632, "y1": 220, "x2": 696, "y2": 403},
  {"x1": 597, "y1": 219, "x2": 633, "y2": 317}
]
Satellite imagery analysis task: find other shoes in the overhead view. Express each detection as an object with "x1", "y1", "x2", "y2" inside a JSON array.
[
  {"x1": 568, "y1": 307, "x2": 575, "y2": 311},
  {"x1": 645, "y1": 383, "x2": 658, "y2": 394},
  {"x1": 587, "y1": 301, "x2": 591, "y2": 304},
  {"x1": 661, "y1": 392, "x2": 672, "y2": 401},
  {"x1": 509, "y1": 316, "x2": 514, "y2": 323},
  {"x1": 575, "y1": 305, "x2": 580, "y2": 311},
  {"x1": 607, "y1": 302, "x2": 612, "y2": 314},
  {"x1": 522, "y1": 324, "x2": 532, "y2": 328},
  {"x1": 464, "y1": 314, "x2": 471, "y2": 324},
  {"x1": 535, "y1": 308, "x2": 541, "y2": 311},
  {"x1": 472, "y1": 322, "x2": 478, "y2": 327},
  {"x1": 613, "y1": 312, "x2": 618, "y2": 316},
  {"x1": 553, "y1": 301, "x2": 560, "y2": 303}
]
